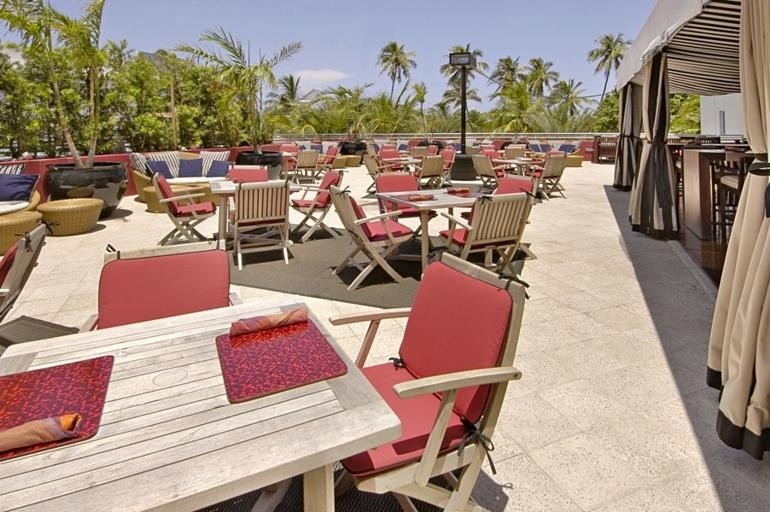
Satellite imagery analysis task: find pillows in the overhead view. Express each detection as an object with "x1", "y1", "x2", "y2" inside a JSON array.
[
  {"x1": 299, "y1": 145, "x2": 306, "y2": 150},
  {"x1": 558, "y1": 144, "x2": 576, "y2": 154},
  {"x1": 311, "y1": 144, "x2": 323, "y2": 154},
  {"x1": 1, "y1": 172, "x2": 39, "y2": 202},
  {"x1": 206, "y1": 160, "x2": 235, "y2": 177},
  {"x1": 177, "y1": 158, "x2": 203, "y2": 177},
  {"x1": 145, "y1": 160, "x2": 173, "y2": 179},
  {"x1": 531, "y1": 144, "x2": 551, "y2": 152}
]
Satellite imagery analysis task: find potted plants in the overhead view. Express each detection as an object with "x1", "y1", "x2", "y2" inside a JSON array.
[
  {"x1": 410, "y1": 81, "x2": 444, "y2": 155},
  {"x1": 1, "y1": 0, "x2": 129, "y2": 220},
  {"x1": 326, "y1": 81, "x2": 375, "y2": 165},
  {"x1": 170, "y1": 25, "x2": 305, "y2": 180},
  {"x1": 501, "y1": 59, "x2": 530, "y2": 150}
]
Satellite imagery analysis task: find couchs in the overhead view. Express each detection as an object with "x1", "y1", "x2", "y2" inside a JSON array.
[
  {"x1": 129, "y1": 150, "x2": 235, "y2": 203},
  {"x1": 530, "y1": 142, "x2": 582, "y2": 155},
  {"x1": 291, "y1": 140, "x2": 340, "y2": 154},
  {"x1": 0, "y1": 165, "x2": 40, "y2": 213}
]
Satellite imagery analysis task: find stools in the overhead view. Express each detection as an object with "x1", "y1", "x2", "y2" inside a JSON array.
[
  {"x1": 333, "y1": 155, "x2": 347, "y2": 168},
  {"x1": 143, "y1": 185, "x2": 191, "y2": 213},
  {"x1": 37, "y1": 198, "x2": 104, "y2": 236},
  {"x1": 0, "y1": 209, "x2": 42, "y2": 256},
  {"x1": 565, "y1": 156, "x2": 584, "y2": 167},
  {"x1": 347, "y1": 155, "x2": 361, "y2": 167}
]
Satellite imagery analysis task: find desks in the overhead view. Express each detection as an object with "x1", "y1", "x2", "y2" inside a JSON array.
[
  {"x1": 280, "y1": 151, "x2": 296, "y2": 181},
  {"x1": 683, "y1": 146, "x2": 753, "y2": 241},
  {"x1": 492, "y1": 156, "x2": 545, "y2": 175},
  {"x1": 0, "y1": 293, "x2": 401, "y2": 512},
  {"x1": 166, "y1": 176, "x2": 227, "y2": 183},
  {"x1": 382, "y1": 158, "x2": 422, "y2": 173},
  {"x1": 376, "y1": 188, "x2": 476, "y2": 279},
  {"x1": 667, "y1": 143, "x2": 749, "y2": 168},
  {"x1": 210, "y1": 178, "x2": 303, "y2": 252},
  {"x1": 0, "y1": 200, "x2": 30, "y2": 214}
]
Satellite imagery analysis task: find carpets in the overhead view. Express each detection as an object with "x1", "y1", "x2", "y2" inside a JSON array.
[
  {"x1": 0, "y1": 315, "x2": 460, "y2": 512},
  {"x1": 230, "y1": 222, "x2": 530, "y2": 309}
]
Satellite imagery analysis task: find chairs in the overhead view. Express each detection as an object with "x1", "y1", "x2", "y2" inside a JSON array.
[
  {"x1": 480, "y1": 150, "x2": 498, "y2": 158},
  {"x1": 414, "y1": 154, "x2": 444, "y2": 190},
  {"x1": 439, "y1": 192, "x2": 529, "y2": 299},
  {"x1": 153, "y1": 172, "x2": 217, "y2": 247},
  {"x1": 529, "y1": 152, "x2": 566, "y2": 191},
  {"x1": 526, "y1": 154, "x2": 567, "y2": 201},
  {"x1": 508, "y1": 143, "x2": 527, "y2": 148},
  {"x1": 504, "y1": 146, "x2": 524, "y2": 157},
  {"x1": 77, "y1": 238, "x2": 242, "y2": 333},
  {"x1": 410, "y1": 146, "x2": 428, "y2": 159},
  {"x1": 427, "y1": 145, "x2": 438, "y2": 155},
  {"x1": 720, "y1": 152, "x2": 753, "y2": 245},
  {"x1": 667, "y1": 136, "x2": 696, "y2": 143},
  {"x1": 315, "y1": 144, "x2": 340, "y2": 178},
  {"x1": 460, "y1": 174, "x2": 537, "y2": 268},
  {"x1": 227, "y1": 164, "x2": 268, "y2": 183},
  {"x1": 328, "y1": 184, "x2": 414, "y2": 292},
  {"x1": 406, "y1": 139, "x2": 418, "y2": 151},
  {"x1": 379, "y1": 148, "x2": 404, "y2": 173},
  {"x1": 329, "y1": 251, "x2": 530, "y2": 512},
  {"x1": 229, "y1": 180, "x2": 290, "y2": 271},
  {"x1": 675, "y1": 145, "x2": 716, "y2": 231},
  {"x1": 478, "y1": 144, "x2": 495, "y2": 151},
  {"x1": 494, "y1": 140, "x2": 504, "y2": 150},
  {"x1": 290, "y1": 167, "x2": 344, "y2": 243},
  {"x1": 381, "y1": 144, "x2": 397, "y2": 150},
  {"x1": 697, "y1": 135, "x2": 720, "y2": 144},
  {"x1": 0, "y1": 218, "x2": 60, "y2": 323},
  {"x1": 290, "y1": 149, "x2": 319, "y2": 185},
  {"x1": 375, "y1": 174, "x2": 438, "y2": 248},
  {"x1": 471, "y1": 153, "x2": 506, "y2": 193},
  {"x1": 425, "y1": 147, "x2": 455, "y2": 189},
  {"x1": 363, "y1": 153, "x2": 392, "y2": 194}
]
